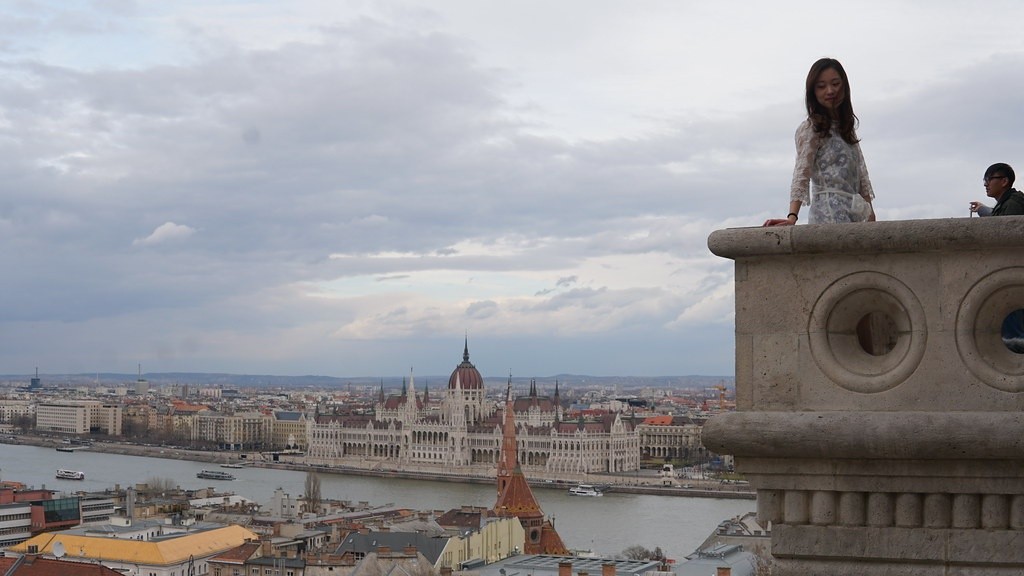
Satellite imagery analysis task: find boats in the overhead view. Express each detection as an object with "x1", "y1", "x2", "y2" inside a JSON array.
[
  {"x1": 56, "y1": 448, "x2": 73, "y2": 452},
  {"x1": 221, "y1": 464, "x2": 243, "y2": 468},
  {"x1": 56, "y1": 469, "x2": 84, "y2": 479},
  {"x1": 197, "y1": 470, "x2": 236, "y2": 481},
  {"x1": 569, "y1": 485, "x2": 603, "y2": 497}
]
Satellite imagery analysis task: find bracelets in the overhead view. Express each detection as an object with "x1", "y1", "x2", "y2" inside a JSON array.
[{"x1": 787, "y1": 213, "x2": 798, "y2": 220}]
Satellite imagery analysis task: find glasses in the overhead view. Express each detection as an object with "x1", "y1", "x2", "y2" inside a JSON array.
[{"x1": 985, "y1": 176, "x2": 1005, "y2": 182}]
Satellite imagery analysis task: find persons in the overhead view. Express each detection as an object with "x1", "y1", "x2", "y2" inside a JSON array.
[
  {"x1": 762, "y1": 57, "x2": 876, "y2": 230},
  {"x1": 970, "y1": 162, "x2": 1024, "y2": 217}
]
